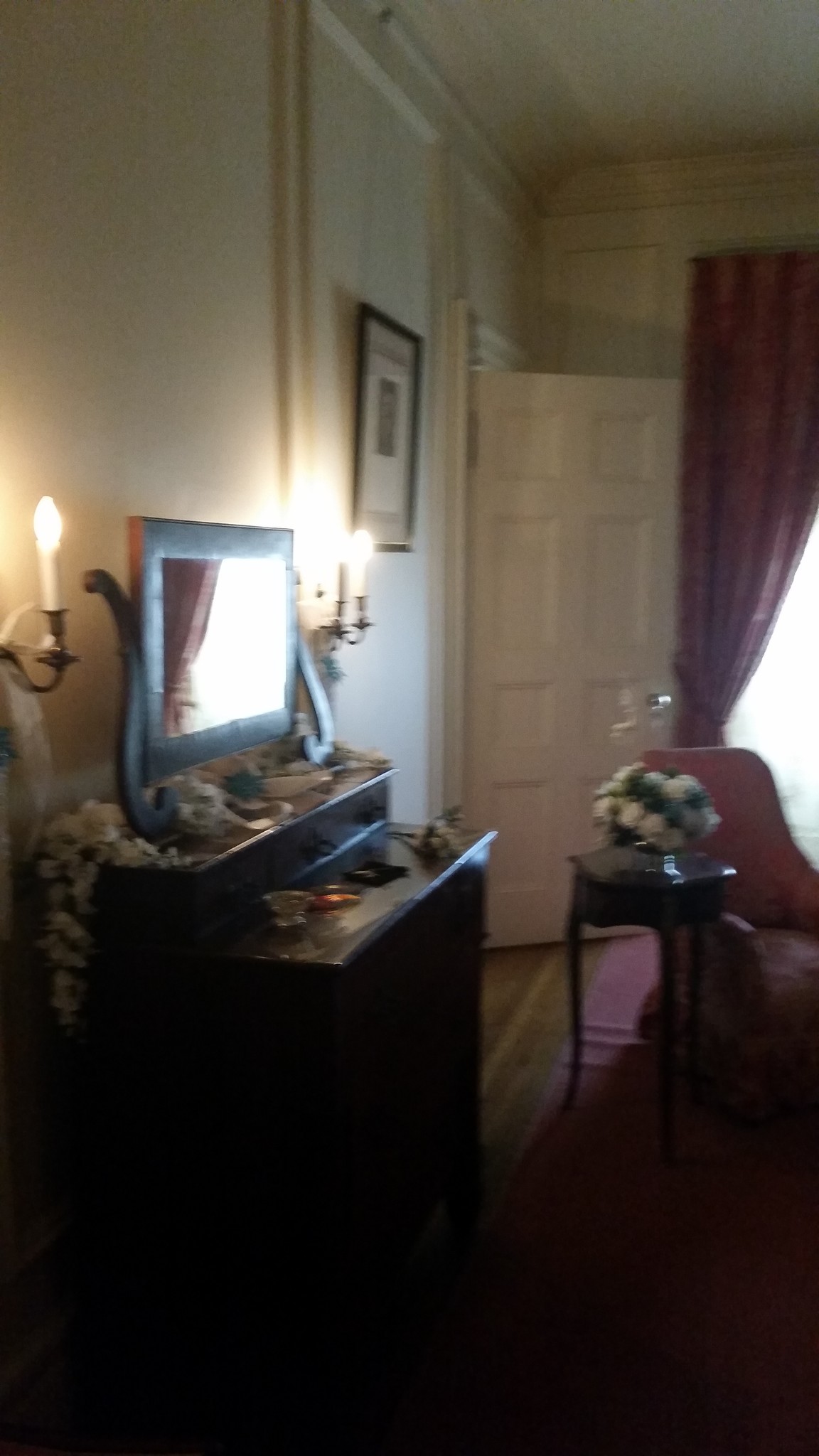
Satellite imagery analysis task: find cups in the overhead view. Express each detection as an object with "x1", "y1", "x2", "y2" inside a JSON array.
[{"x1": 256, "y1": 889, "x2": 311, "y2": 930}]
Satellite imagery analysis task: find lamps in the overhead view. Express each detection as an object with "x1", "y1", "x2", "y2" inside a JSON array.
[
  {"x1": 1, "y1": 496, "x2": 81, "y2": 695},
  {"x1": 311, "y1": 528, "x2": 378, "y2": 644}
]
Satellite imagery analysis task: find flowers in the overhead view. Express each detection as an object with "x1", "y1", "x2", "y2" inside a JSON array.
[{"x1": 591, "y1": 760, "x2": 724, "y2": 860}]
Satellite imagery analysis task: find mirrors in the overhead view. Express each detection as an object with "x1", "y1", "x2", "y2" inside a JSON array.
[{"x1": 81, "y1": 517, "x2": 337, "y2": 843}]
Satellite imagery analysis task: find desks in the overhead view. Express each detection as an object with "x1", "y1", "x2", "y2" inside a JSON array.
[{"x1": 562, "y1": 846, "x2": 739, "y2": 1139}]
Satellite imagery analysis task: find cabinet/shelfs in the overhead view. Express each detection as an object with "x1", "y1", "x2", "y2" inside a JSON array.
[{"x1": 8, "y1": 741, "x2": 499, "y2": 1456}]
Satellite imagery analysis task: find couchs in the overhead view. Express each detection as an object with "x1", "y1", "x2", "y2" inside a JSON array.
[{"x1": 631, "y1": 745, "x2": 819, "y2": 1128}]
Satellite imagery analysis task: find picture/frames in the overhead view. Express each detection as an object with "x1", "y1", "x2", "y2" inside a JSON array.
[{"x1": 351, "y1": 300, "x2": 424, "y2": 555}]
